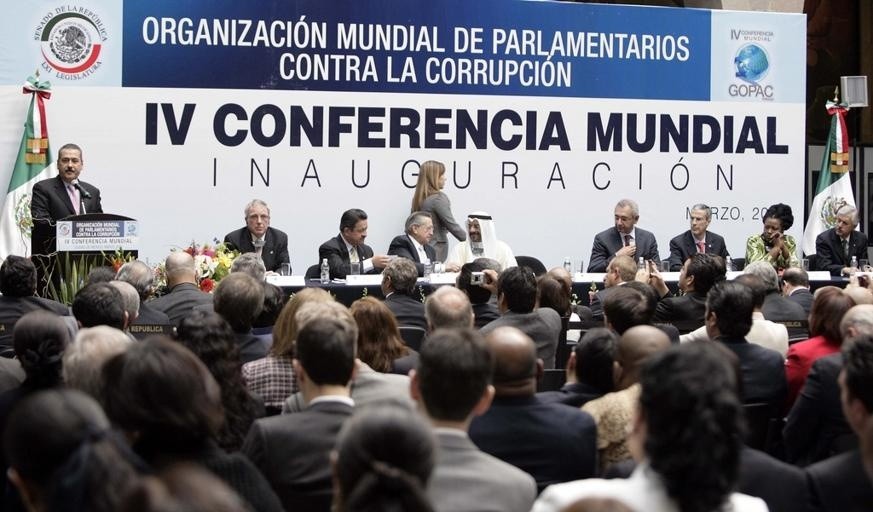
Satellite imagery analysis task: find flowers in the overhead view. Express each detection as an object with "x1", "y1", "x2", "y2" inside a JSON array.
[{"x1": 98, "y1": 236, "x2": 242, "y2": 295}]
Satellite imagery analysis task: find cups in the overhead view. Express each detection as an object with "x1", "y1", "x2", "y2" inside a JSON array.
[
  {"x1": 280, "y1": 262, "x2": 290, "y2": 276},
  {"x1": 660, "y1": 260, "x2": 669, "y2": 273},
  {"x1": 349, "y1": 263, "x2": 360, "y2": 275},
  {"x1": 801, "y1": 259, "x2": 809, "y2": 271},
  {"x1": 859, "y1": 259, "x2": 868, "y2": 271}
]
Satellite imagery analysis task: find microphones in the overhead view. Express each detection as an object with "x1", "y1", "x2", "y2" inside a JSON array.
[{"x1": 70, "y1": 179, "x2": 92, "y2": 198}]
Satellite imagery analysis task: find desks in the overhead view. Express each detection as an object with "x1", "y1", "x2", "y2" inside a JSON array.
[{"x1": 279, "y1": 269, "x2": 850, "y2": 310}]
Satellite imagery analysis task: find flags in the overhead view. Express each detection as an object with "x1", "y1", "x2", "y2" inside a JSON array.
[
  {"x1": 0, "y1": 78, "x2": 58, "y2": 271},
  {"x1": 801, "y1": 101, "x2": 860, "y2": 257}
]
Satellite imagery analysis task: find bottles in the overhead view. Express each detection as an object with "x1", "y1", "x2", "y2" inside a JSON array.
[
  {"x1": 424, "y1": 258, "x2": 432, "y2": 283},
  {"x1": 562, "y1": 257, "x2": 571, "y2": 274},
  {"x1": 723, "y1": 255, "x2": 732, "y2": 276},
  {"x1": 319, "y1": 257, "x2": 329, "y2": 284},
  {"x1": 849, "y1": 255, "x2": 858, "y2": 281},
  {"x1": 636, "y1": 256, "x2": 645, "y2": 272}
]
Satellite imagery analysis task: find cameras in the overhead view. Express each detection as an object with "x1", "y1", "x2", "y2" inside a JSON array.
[{"x1": 470, "y1": 272, "x2": 489, "y2": 285}]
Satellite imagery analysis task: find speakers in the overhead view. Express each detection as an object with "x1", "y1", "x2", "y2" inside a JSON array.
[{"x1": 841, "y1": 75, "x2": 868, "y2": 108}]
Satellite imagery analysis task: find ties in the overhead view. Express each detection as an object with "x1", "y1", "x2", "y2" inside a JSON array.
[
  {"x1": 418, "y1": 244, "x2": 427, "y2": 263},
  {"x1": 842, "y1": 239, "x2": 846, "y2": 255},
  {"x1": 624, "y1": 235, "x2": 630, "y2": 246},
  {"x1": 697, "y1": 242, "x2": 704, "y2": 254},
  {"x1": 256, "y1": 239, "x2": 262, "y2": 256},
  {"x1": 69, "y1": 186, "x2": 79, "y2": 215},
  {"x1": 350, "y1": 248, "x2": 358, "y2": 263}
]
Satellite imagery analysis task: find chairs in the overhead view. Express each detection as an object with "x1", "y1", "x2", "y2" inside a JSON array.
[
  {"x1": 741, "y1": 401, "x2": 769, "y2": 451},
  {"x1": 395, "y1": 325, "x2": 425, "y2": 353},
  {"x1": 539, "y1": 368, "x2": 566, "y2": 392}
]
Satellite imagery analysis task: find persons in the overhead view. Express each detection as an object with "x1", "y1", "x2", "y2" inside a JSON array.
[
  {"x1": 587, "y1": 198, "x2": 663, "y2": 273},
  {"x1": 669, "y1": 204, "x2": 737, "y2": 271},
  {"x1": 411, "y1": 160, "x2": 465, "y2": 263},
  {"x1": 0, "y1": 253, "x2": 872, "y2": 511},
  {"x1": 222, "y1": 199, "x2": 291, "y2": 275},
  {"x1": 317, "y1": 210, "x2": 394, "y2": 279},
  {"x1": 30, "y1": 143, "x2": 103, "y2": 261},
  {"x1": 448, "y1": 211, "x2": 519, "y2": 273},
  {"x1": 387, "y1": 212, "x2": 459, "y2": 272},
  {"x1": 744, "y1": 204, "x2": 801, "y2": 269},
  {"x1": 817, "y1": 204, "x2": 873, "y2": 277}
]
